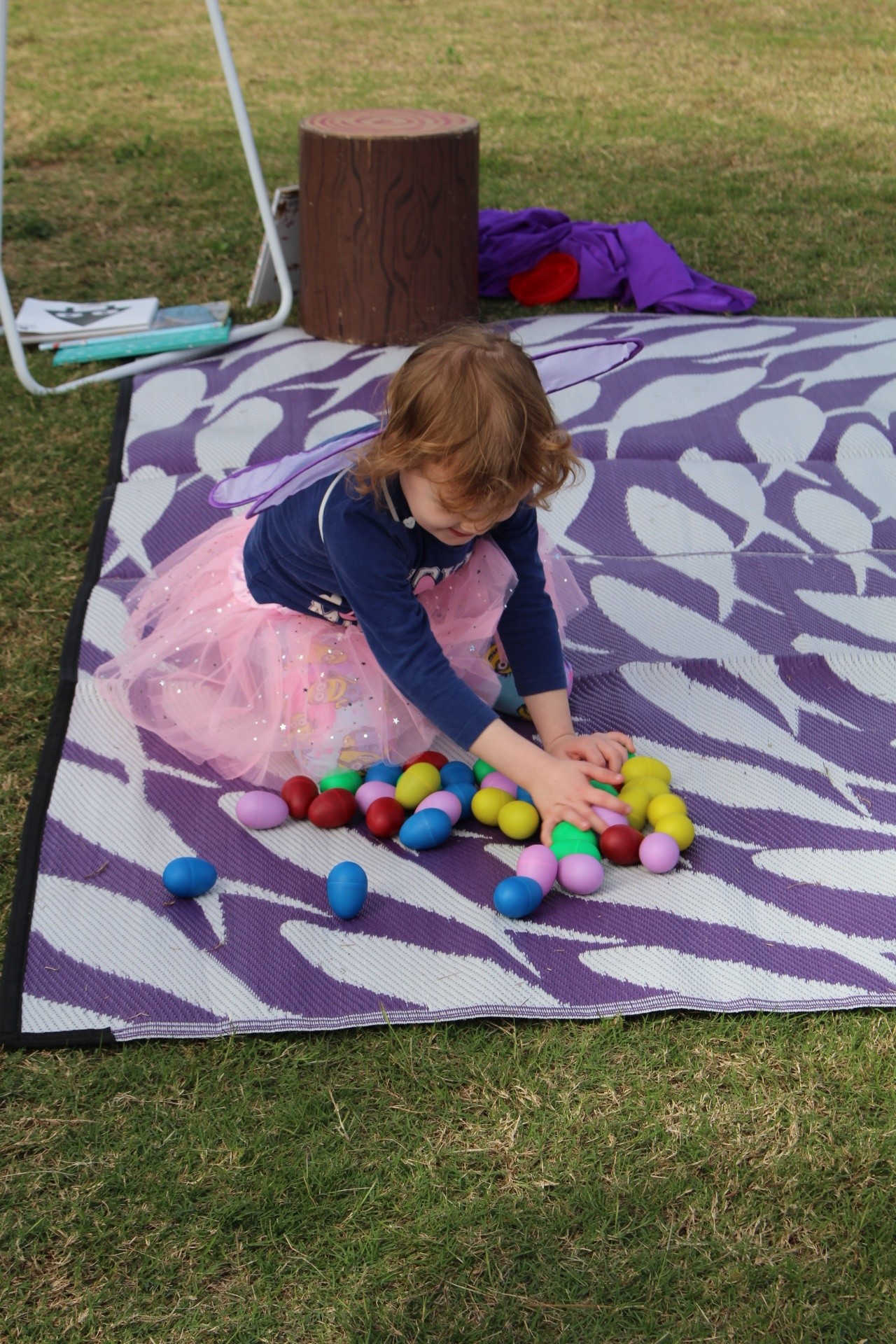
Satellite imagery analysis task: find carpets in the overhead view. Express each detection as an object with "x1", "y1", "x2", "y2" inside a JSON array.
[{"x1": 0, "y1": 315, "x2": 896, "y2": 1048}]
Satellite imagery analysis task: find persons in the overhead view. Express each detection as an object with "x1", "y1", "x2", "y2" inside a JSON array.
[{"x1": 91, "y1": 323, "x2": 636, "y2": 846}]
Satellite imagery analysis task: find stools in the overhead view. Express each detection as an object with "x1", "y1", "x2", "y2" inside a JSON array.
[{"x1": 297, "y1": 106, "x2": 483, "y2": 352}]
